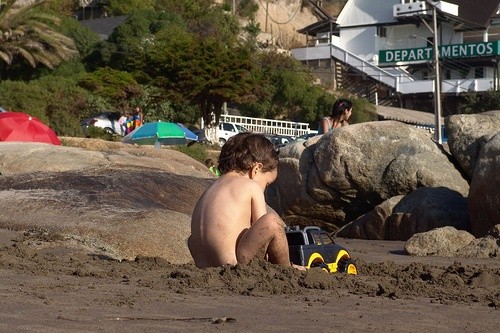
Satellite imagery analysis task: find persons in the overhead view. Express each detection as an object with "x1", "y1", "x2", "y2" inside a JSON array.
[
  {"x1": 117, "y1": 103, "x2": 143, "y2": 138},
  {"x1": 318, "y1": 97, "x2": 353, "y2": 135},
  {"x1": 187, "y1": 131, "x2": 306, "y2": 271},
  {"x1": 203, "y1": 157, "x2": 219, "y2": 177}
]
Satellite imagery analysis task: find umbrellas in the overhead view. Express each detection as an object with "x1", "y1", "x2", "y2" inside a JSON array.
[
  {"x1": 0, "y1": 111, "x2": 61, "y2": 145},
  {"x1": 123, "y1": 118, "x2": 198, "y2": 150}
]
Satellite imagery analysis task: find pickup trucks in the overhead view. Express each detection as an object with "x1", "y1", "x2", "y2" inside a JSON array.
[{"x1": 284, "y1": 225, "x2": 358, "y2": 275}]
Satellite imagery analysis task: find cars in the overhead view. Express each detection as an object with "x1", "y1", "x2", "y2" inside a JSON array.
[
  {"x1": 210, "y1": 122, "x2": 251, "y2": 149},
  {"x1": 81, "y1": 110, "x2": 128, "y2": 138},
  {"x1": 264, "y1": 133, "x2": 294, "y2": 149}
]
